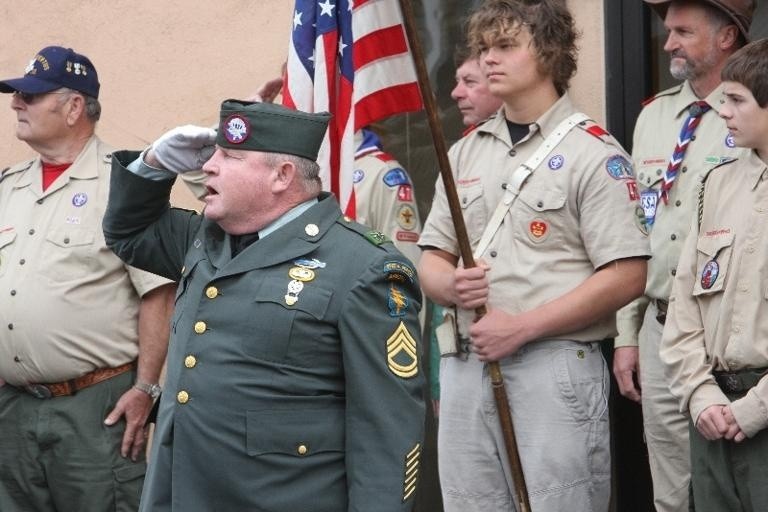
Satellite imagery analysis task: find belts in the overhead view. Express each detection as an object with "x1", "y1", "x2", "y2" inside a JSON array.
[
  {"x1": 26, "y1": 363, "x2": 133, "y2": 401},
  {"x1": 716, "y1": 372, "x2": 758, "y2": 395}
]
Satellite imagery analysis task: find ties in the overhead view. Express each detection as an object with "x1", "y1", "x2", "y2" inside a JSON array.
[
  {"x1": 232, "y1": 233, "x2": 260, "y2": 260},
  {"x1": 657, "y1": 100, "x2": 710, "y2": 206}
]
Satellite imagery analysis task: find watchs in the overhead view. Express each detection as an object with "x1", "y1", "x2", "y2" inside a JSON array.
[{"x1": 135, "y1": 380, "x2": 163, "y2": 400}]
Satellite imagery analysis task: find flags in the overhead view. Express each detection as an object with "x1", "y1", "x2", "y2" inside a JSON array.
[{"x1": 283, "y1": 0, "x2": 428, "y2": 224}]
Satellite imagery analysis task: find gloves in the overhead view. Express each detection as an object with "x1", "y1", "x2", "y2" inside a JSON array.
[{"x1": 151, "y1": 125, "x2": 218, "y2": 176}]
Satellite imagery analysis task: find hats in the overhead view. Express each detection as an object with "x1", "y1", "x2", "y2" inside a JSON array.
[
  {"x1": 216, "y1": 98, "x2": 334, "y2": 162},
  {"x1": 649, "y1": 0, "x2": 757, "y2": 46},
  {"x1": 0, "y1": 45, "x2": 100, "y2": 99}
]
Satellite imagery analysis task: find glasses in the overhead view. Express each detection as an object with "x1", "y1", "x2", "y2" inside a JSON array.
[{"x1": 13, "y1": 89, "x2": 74, "y2": 105}]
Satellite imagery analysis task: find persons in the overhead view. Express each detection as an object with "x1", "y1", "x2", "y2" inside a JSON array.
[
  {"x1": 0, "y1": 46, "x2": 175, "y2": 512},
  {"x1": 416, "y1": 0, "x2": 653, "y2": 512},
  {"x1": 177, "y1": 56, "x2": 429, "y2": 354},
  {"x1": 100, "y1": 96, "x2": 427, "y2": 512},
  {"x1": 659, "y1": 34, "x2": 768, "y2": 512},
  {"x1": 451, "y1": 36, "x2": 503, "y2": 126},
  {"x1": 611, "y1": 0, "x2": 756, "y2": 512}
]
